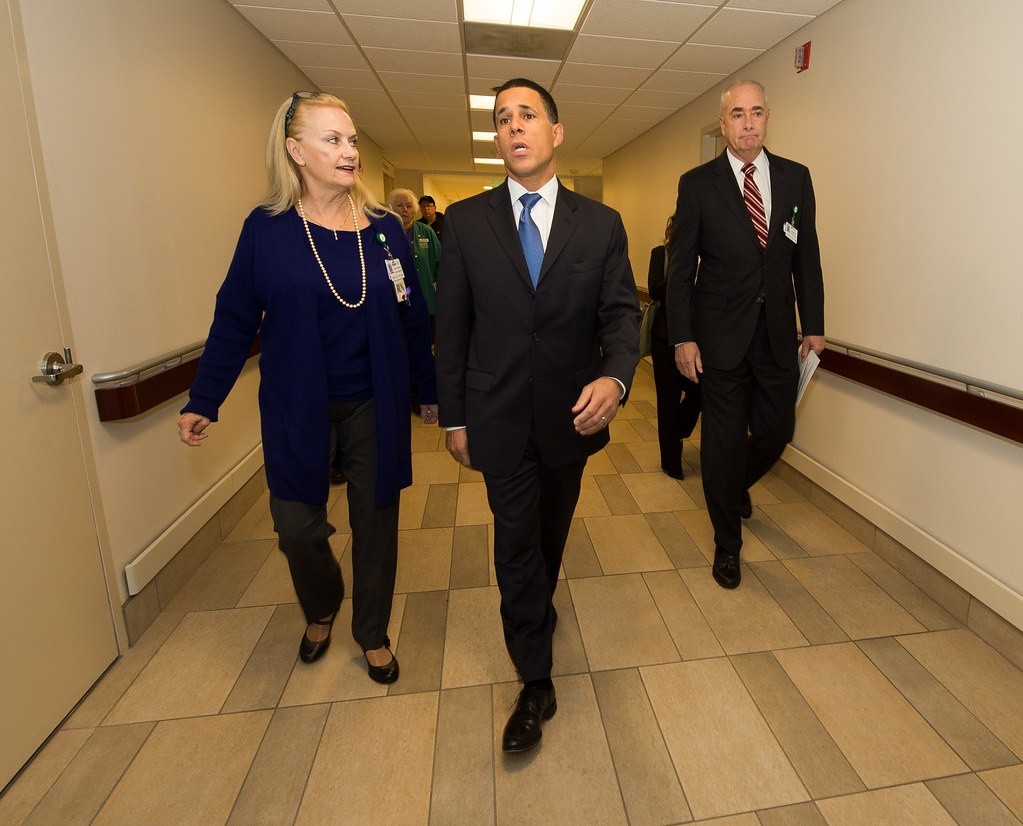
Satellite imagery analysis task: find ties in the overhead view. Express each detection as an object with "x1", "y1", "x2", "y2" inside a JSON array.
[
  {"x1": 519, "y1": 193, "x2": 545, "y2": 290},
  {"x1": 741, "y1": 162, "x2": 768, "y2": 251}
]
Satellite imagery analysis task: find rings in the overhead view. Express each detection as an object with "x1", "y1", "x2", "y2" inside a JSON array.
[
  {"x1": 177, "y1": 429, "x2": 182, "y2": 433},
  {"x1": 602, "y1": 416, "x2": 608, "y2": 422}
]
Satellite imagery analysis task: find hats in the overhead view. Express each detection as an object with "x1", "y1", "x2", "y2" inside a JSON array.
[{"x1": 418, "y1": 196, "x2": 434, "y2": 204}]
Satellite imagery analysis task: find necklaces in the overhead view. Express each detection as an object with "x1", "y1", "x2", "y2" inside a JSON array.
[{"x1": 299, "y1": 194, "x2": 366, "y2": 307}]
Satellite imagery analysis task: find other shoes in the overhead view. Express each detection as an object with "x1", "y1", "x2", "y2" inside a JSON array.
[
  {"x1": 364, "y1": 636, "x2": 399, "y2": 684},
  {"x1": 299, "y1": 605, "x2": 340, "y2": 663},
  {"x1": 329, "y1": 465, "x2": 350, "y2": 484},
  {"x1": 663, "y1": 466, "x2": 684, "y2": 480}
]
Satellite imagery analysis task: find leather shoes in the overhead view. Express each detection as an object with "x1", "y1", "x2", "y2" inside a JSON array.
[
  {"x1": 741, "y1": 491, "x2": 752, "y2": 519},
  {"x1": 502, "y1": 684, "x2": 557, "y2": 752},
  {"x1": 713, "y1": 546, "x2": 742, "y2": 588}
]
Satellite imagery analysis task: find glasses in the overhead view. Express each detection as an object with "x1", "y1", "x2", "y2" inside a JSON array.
[{"x1": 283, "y1": 91, "x2": 319, "y2": 137}]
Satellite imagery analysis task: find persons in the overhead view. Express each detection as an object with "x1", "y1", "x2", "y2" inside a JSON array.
[
  {"x1": 388, "y1": 189, "x2": 444, "y2": 417},
  {"x1": 647, "y1": 79, "x2": 825, "y2": 589},
  {"x1": 177, "y1": 91, "x2": 439, "y2": 684},
  {"x1": 433, "y1": 78, "x2": 643, "y2": 752}
]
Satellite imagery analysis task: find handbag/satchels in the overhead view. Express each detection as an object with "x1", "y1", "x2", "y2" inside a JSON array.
[{"x1": 638, "y1": 246, "x2": 669, "y2": 357}]
